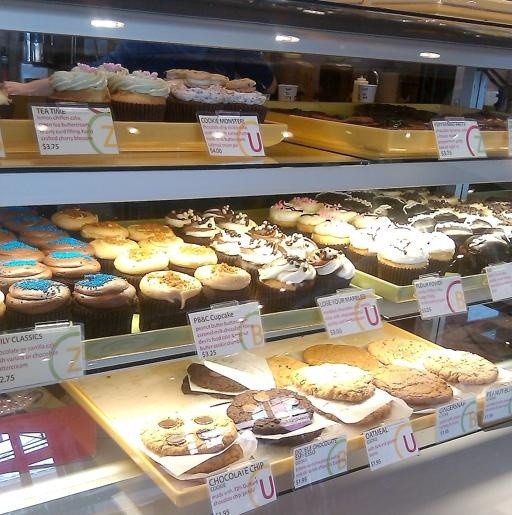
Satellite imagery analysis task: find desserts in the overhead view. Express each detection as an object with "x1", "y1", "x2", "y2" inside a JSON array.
[{"x1": 0, "y1": 187, "x2": 512, "y2": 339}]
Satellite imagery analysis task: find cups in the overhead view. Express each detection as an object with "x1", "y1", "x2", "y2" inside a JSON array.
[
  {"x1": 360, "y1": 85, "x2": 377, "y2": 102},
  {"x1": 277, "y1": 84, "x2": 299, "y2": 102}
]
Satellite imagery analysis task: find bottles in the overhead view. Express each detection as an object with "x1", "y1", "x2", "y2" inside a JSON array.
[{"x1": 352, "y1": 76, "x2": 369, "y2": 103}]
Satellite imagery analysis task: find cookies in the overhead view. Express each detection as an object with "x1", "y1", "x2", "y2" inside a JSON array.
[{"x1": 141, "y1": 339, "x2": 499, "y2": 485}]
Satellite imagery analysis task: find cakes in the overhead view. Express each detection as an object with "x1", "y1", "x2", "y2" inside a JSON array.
[{"x1": 1, "y1": 63, "x2": 268, "y2": 124}]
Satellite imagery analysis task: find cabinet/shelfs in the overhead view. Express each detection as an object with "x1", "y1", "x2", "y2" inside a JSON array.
[{"x1": 1, "y1": 0, "x2": 512, "y2": 515}]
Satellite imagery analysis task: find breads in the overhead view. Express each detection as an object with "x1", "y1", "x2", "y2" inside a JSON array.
[
  {"x1": 462, "y1": 321, "x2": 512, "y2": 358},
  {"x1": 269, "y1": 103, "x2": 512, "y2": 131}
]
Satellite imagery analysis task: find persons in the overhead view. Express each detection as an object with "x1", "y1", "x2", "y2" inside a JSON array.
[{"x1": 5, "y1": 37, "x2": 277, "y2": 97}]
